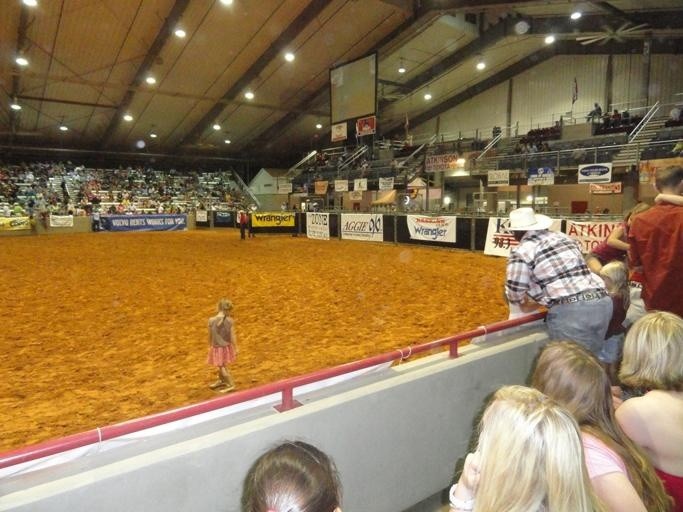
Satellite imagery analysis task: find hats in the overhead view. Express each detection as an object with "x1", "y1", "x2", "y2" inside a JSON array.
[{"x1": 505, "y1": 208, "x2": 553, "y2": 231}]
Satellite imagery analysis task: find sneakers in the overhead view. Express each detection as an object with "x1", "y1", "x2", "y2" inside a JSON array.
[
  {"x1": 210, "y1": 380, "x2": 226, "y2": 388},
  {"x1": 221, "y1": 386, "x2": 234, "y2": 392}
]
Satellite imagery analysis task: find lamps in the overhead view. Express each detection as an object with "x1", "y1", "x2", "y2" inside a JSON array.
[
  {"x1": 10, "y1": 94, "x2": 22, "y2": 111},
  {"x1": 15, "y1": 42, "x2": 32, "y2": 67}
]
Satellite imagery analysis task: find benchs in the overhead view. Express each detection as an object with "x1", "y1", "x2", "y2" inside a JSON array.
[{"x1": 0, "y1": 166, "x2": 255, "y2": 217}]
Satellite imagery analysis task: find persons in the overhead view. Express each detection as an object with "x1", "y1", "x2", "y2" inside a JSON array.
[
  {"x1": 584, "y1": 164, "x2": 681, "y2": 340},
  {"x1": 279, "y1": 100, "x2": 683, "y2": 239},
  {"x1": 529, "y1": 337, "x2": 677, "y2": 511},
  {"x1": 206, "y1": 299, "x2": 237, "y2": 393},
  {"x1": 237, "y1": 441, "x2": 341, "y2": 511},
  {"x1": 503, "y1": 207, "x2": 626, "y2": 398},
  {"x1": 445, "y1": 383, "x2": 611, "y2": 512},
  {"x1": 0, "y1": 157, "x2": 261, "y2": 240},
  {"x1": 603, "y1": 310, "x2": 683, "y2": 512}
]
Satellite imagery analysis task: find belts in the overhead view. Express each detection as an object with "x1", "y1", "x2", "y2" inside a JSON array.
[
  {"x1": 632, "y1": 282, "x2": 642, "y2": 288},
  {"x1": 558, "y1": 292, "x2": 608, "y2": 302}
]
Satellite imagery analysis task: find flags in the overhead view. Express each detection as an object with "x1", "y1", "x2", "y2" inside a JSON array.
[{"x1": 571, "y1": 77, "x2": 578, "y2": 105}]
